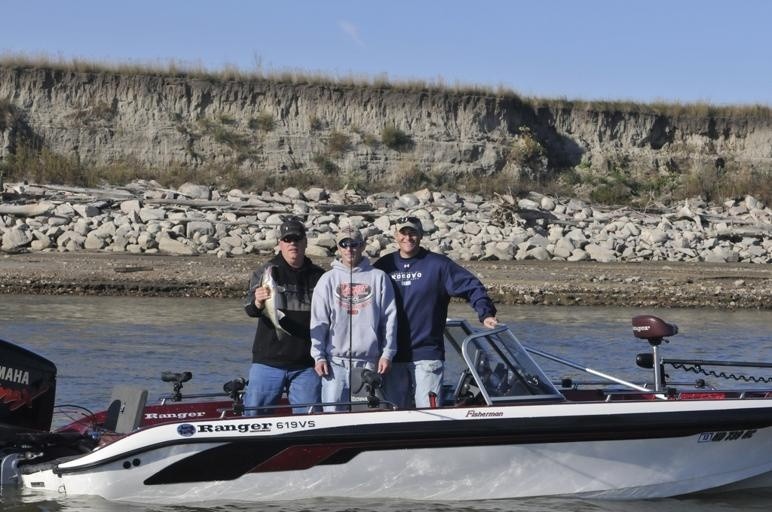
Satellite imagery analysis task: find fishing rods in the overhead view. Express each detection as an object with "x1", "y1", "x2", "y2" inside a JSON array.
[{"x1": 445, "y1": 316, "x2": 672, "y2": 401}]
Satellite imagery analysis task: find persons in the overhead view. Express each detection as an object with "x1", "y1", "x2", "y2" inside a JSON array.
[
  {"x1": 373, "y1": 214, "x2": 500, "y2": 411},
  {"x1": 313, "y1": 227, "x2": 399, "y2": 411},
  {"x1": 243, "y1": 220, "x2": 327, "y2": 418}
]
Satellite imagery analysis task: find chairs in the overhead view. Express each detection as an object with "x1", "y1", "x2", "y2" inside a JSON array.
[{"x1": 349, "y1": 368, "x2": 391, "y2": 412}]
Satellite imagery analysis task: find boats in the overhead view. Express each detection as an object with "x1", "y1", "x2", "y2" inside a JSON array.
[{"x1": 0, "y1": 318, "x2": 772, "y2": 504}]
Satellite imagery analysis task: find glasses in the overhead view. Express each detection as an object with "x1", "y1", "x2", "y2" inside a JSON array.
[
  {"x1": 396, "y1": 217, "x2": 420, "y2": 224},
  {"x1": 280, "y1": 236, "x2": 301, "y2": 242},
  {"x1": 340, "y1": 240, "x2": 358, "y2": 247}
]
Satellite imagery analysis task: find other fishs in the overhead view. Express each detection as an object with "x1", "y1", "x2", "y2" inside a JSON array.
[{"x1": 261, "y1": 265, "x2": 292, "y2": 342}]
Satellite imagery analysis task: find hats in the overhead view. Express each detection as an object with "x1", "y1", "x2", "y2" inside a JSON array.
[
  {"x1": 396, "y1": 215, "x2": 422, "y2": 233},
  {"x1": 280, "y1": 221, "x2": 305, "y2": 238},
  {"x1": 336, "y1": 227, "x2": 363, "y2": 244}
]
